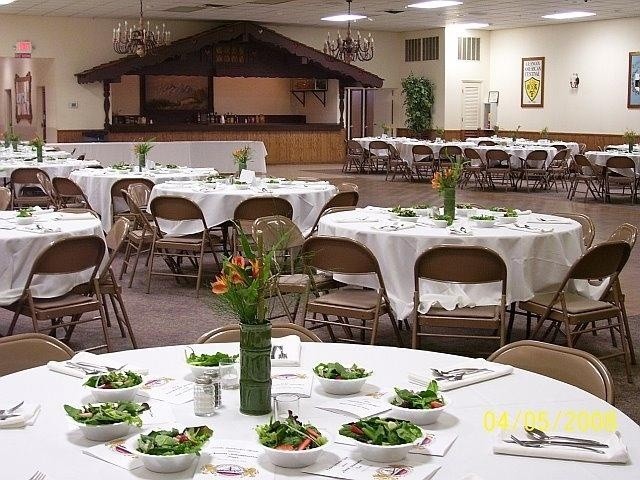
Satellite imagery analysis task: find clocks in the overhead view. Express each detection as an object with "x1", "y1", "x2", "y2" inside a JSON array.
[{"x1": 488, "y1": 91, "x2": 499, "y2": 103}]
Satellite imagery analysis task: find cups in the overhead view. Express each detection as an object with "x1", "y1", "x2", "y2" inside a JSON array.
[
  {"x1": 273, "y1": 394, "x2": 299, "y2": 422},
  {"x1": 219, "y1": 361, "x2": 239, "y2": 389}
]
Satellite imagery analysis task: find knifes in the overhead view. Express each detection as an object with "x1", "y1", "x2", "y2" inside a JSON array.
[
  {"x1": 65, "y1": 365, "x2": 107, "y2": 373},
  {"x1": 501, "y1": 439, "x2": 609, "y2": 449}
]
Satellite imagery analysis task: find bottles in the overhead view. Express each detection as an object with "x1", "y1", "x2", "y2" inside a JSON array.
[
  {"x1": 197, "y1": 111, "x2": 265, "y2": 124},
  {"x1": 114, "y1": 114, "x2": 154, "y2": 125},
  {"x1": 201, "y1": 368, "x2": 222, "y2": 408},
  {"x1": 194, "y1": 375, "x2": 216, "y2": 417}
]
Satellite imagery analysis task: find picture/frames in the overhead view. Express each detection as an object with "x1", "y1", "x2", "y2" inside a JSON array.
[
  {"x1": 139, "y1": 75, "x2": 214, "y2": 114},
  {"x1": 626, "y1": 52, "x2": 640, "y2": 109},
  {"x1": 521, "y1": 58, "x2": 545, "y2": 107},
  {"x1": 14, "y1": 71, "x2": 33, "y2": 124}
]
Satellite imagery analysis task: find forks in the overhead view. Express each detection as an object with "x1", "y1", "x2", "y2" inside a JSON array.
[
  {"x1": 510, "y1": 433, "x2": 605, "y2": 456},
  {"x1": 29, "y1": 470, "x2": 46, "y2": 480},
  {"x1": 76, "y1": 362, "x2": 128, "y2": 372}
]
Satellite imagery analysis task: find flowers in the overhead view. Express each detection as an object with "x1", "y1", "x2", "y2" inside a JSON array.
[{"x1": 622, "y1": 128, "x2": 638, "y2": 142}]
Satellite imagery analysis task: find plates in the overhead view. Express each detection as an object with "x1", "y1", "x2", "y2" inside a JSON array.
[
  {"x1": 124, "y1": 431, "x2": 198, "y2": 472},
  {"x1": 256, "y1": 434, "x2": 332, "y2": 468},
  {"x1": 190, "y1": 359, "x2": 236, "y2": 378},
  {"x1": 313, "y1": 367, "x2": 375, "y2": 396},
  {"x1": 76, "y1": 403, "x2": 135, "y2": 440},
  {"x1": 386, "y1": 395, "x2": 447, "y2": 426},
  {"x1": 350, "y1": 425, "x2": 426, "y2": 463},
  {"x1": 82, "y1": 373, "x2": 144, "y2": 401}
]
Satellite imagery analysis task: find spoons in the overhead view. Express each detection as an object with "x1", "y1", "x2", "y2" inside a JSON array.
[
  {"x1": 523, "y1": 425, "x2": 600, "y2": 445},
  {"x1": 66, "y1": 361, "x2": 99, "y2": 376}
]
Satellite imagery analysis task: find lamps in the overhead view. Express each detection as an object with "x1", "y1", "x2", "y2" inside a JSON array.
[
  {"x1": 323, "y1": 0, "x2": 374, "y2": 66},
  {"x1": 112, "y1": 0, "x2": 171, "y2": 58}
]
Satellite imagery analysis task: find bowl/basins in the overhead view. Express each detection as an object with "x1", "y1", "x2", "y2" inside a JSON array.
[
  {"x1": 111, "y1": 163, "x2": 178, "y2": 174},
  {"x1": 14, "y1": 216, "x2": 36, "y2": 225},
  {"x1": 204, "y1": 175, "x2": 280, "y2": 190},
  {"x1": 388, "y1": 207, "x2": 520, "y2": 228}
]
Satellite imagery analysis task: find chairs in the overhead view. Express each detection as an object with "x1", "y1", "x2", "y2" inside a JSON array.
[{"x1": 341, "y1": 137, "x2": 640, "y2": 206}]
